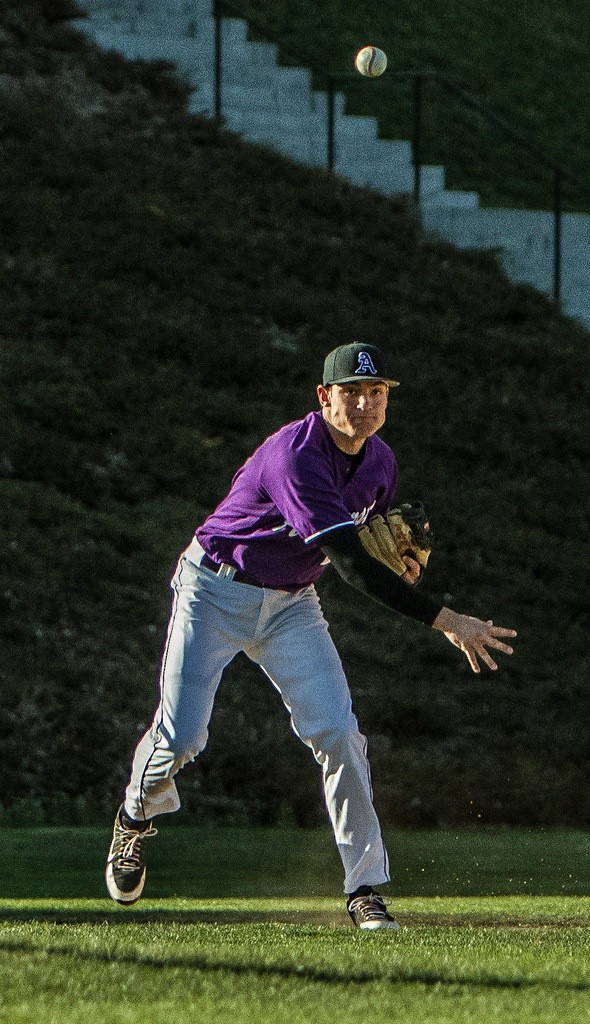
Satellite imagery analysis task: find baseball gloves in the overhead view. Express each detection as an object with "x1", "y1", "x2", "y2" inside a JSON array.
[{"x1": 358, "y1": 497, "x2": 437, "y2": 591}]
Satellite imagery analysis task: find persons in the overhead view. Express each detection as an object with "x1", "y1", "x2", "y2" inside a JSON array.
[{"x1": 107, "y1": 344, "x2": 518, "y2": 930}]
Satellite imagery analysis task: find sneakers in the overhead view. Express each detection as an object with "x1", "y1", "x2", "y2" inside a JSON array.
[
  {"x1": 346, "y1": 891, "x2": 401, "y2": 930},
  {"x1": 105, "y1": 802, "x2": 158, "y2": 908}
]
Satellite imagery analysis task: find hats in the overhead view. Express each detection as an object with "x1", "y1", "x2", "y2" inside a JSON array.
[{"x1": 322, "y1": 341, "x2": 400, "y2": 388}]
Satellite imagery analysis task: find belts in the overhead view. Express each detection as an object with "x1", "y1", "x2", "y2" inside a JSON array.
[{"x1": 199, "y1": 555, "x2": 312, "y2": 593}]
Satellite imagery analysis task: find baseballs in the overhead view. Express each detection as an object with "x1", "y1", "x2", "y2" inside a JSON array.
[{"x1": 354, "y1": 45, "x2": 388, "y2": 78}]
